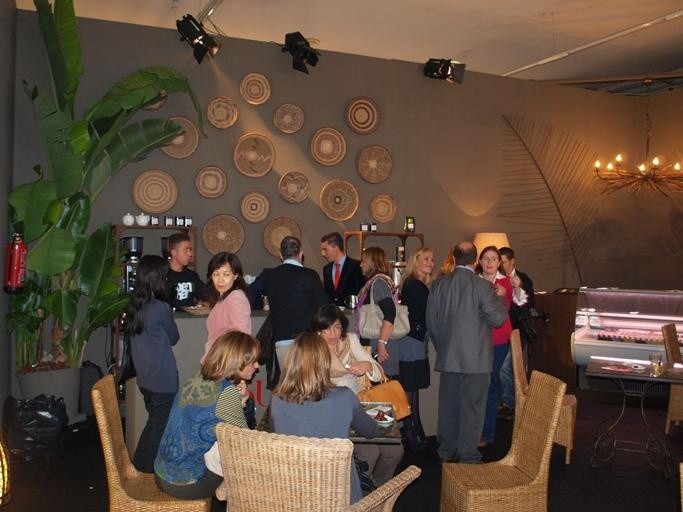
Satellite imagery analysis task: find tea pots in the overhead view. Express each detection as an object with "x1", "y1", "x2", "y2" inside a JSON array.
[{"x1": 120, "y1": 212, "x2": 150, "y2": 227}]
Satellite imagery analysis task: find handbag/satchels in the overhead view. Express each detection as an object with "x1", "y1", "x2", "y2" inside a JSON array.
[
  {"x1": 254, "y1": 317, "x2": 273, "y2": 361},
  {"x1": 359, "y1": 303, "x2": 410, "y2": 339},
  {"x1": 117, "y1": 333, "x2": 137, "y2": 385},
  {"x1": 356, "y1": 380, "x2": 413, "y2": 421},
  {"x1": 406, "y1": 319, "x2": 428, "y2": 342}
]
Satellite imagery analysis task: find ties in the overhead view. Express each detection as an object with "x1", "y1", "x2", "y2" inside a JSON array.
[{"x1": 334, "y1": 263, "x2": 340, "y2": 290}]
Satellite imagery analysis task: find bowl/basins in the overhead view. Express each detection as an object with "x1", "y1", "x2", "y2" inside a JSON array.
[{"x1": 372, "y1": 413, "x2": 395, "y2": 428}]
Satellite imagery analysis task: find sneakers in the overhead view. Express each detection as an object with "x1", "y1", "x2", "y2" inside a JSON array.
[
  {"x1": 479, "y1": 440, "x2": 491, "y2": 447},
  {"x1": 498, "y1": 404, "x2": 515, "y2": 421}
]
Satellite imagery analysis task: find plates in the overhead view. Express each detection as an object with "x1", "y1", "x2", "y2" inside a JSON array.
[
  {"x1": 318, "y1": 178, "x2": 359, "y2": 222},
  {"x1": 263, "y1": 217, "x2": 302, "y2": 258},
  {"x1": 208, "y1": 96, "x2": 239, "y2": 129},
  {"x1": 369, "y1": 195, "x2": 396, "y2": 224},
  {"x1": 240, "y1": 192, "x2": 271, "y2": 223},
  {"x1": 630, "y1": 364, "x2": 647, "y2": 371},
  {"x1": 239, "y1": 73, "x2": 271, "y2": 107},
  {"x1": 134, "y1": 170, "x2": 178, "y2": 215},
  {"x1": 233, "y1": 133, "x2": 275, "y2": 178},
  {"x1": 272, "y1": 103, "x2": 306, "y2": 135},
  {"x1": 160, "y1": 117, "x2": 199, "y2": 160},
  {"x1": 278, "y1": 171, "x2": 311, "y2": 204},
  {"x1": 358, "y1": 144, "x2": 393, "y2": 186},
  {"x1": 310, "y1": 128, "x2": 348, "y2": 167},
  {"x1": 140, "y1": 90, "x2": 167, "y2": 111},
  {"x1": 195, "y1": 166, "x2": 227, "y2": 199},
  {"x1": 201, "y1": 214, "x2": 245, "y2": 256},
  {"x1": 345, "y1": 96, "x2": 381, "y2": 136}
]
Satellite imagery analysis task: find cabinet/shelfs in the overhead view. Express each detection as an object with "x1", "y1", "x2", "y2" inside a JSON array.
[
  {"x1": 115, "y1": 224, "x2": 198, "y2": 290},
  {"x1": 341, "y1": 225, "x2": 429, "y2": 282},
  {"x1": 527, "y1": 286, "x2": 683, "y2": 398}
]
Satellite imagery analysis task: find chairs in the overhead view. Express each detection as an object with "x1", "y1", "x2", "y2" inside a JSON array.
[
  {"x1": 357, "y1": 346, "x2": 372, "y2": 390},
  {"x1": 510, "y1": 327, "x2": 578, "y2": 467},
  {"x1": 90, "y1": 371, "x2": 212, "y2": 512},
  {"x1": 659, "y1": 321, "x2": 682, "y2": 434},
  {"x1": 275, "y1": 339, "x2": 296, "y2": 373},
  {"x1": 437, "y1": 368, "x2": 567, "y2": 511},
  {"x1": 214, "y1": 422, "x2": 422, "y2": 512}
]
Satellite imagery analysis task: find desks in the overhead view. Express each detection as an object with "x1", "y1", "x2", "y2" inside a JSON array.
[
  {"x1": 254, "y1": 401, "x2": 404, "y2": 449},
  {"x1": 584, "y1": 355, "x2": 682, "y2": 478}
]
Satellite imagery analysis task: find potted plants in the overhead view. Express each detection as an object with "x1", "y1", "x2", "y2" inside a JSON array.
[{"x1": 0, "y1": 0, "x2": 210, "y2": 424}]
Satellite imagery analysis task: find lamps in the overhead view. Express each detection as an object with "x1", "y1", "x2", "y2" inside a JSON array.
[
  {"x1": 421, "y1": 51, "x2": 467, "y2": 91},
  {"x1": 175, "y1": 1, "x2": 227, "y2": 63},
  {"x1": 278, "y1": 28, "x2": 319, "y2": 75},
  {"x1": 589, "y1": 80, "x2": 683, "y2": 209}
]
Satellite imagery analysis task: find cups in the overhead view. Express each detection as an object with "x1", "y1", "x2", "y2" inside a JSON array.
[
  {"x1": 344, "y1": 294, "x2": 359, "y2": 309},
  {"x1": 648, "y1": 352, "x2": 662, "y2": 379}
]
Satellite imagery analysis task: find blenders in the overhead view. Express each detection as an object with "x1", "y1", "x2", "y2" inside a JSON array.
[{"x1": 120, "y1": 236, "x2": 142, "y2": 293}]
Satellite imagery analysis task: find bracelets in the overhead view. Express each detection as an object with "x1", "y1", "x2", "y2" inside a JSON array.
[{"x1": 378, "y1": 338, "x2": 388, "y2": 344}]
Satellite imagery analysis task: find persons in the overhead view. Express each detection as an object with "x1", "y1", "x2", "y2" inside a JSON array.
[
  {"x1": 201, "y1": 253, "x2": 251, "y2": 363},
  {"x1": 153, "y1": 332, "x2": 263, "y2": 500},
  {"x1": 314, "y1": 304, "x2": 385, "y2": 394},
  {"x1": 155, "y1": 234, "x2": 210, "y2": 311},
  {"x1": 321, "y1": 234, "x2": 367, "y2": 305},
  {"x1": 249, "y1": 236, "x2": 331, "y2": 390},
  {"x1": 125, "y1": 254, "x2": 180, "y2": 475},
  {"x1": 399, "y1": 233, "x2": 535, "y2": 463},
  {"x1": 271, "y1": 332, "x2": 380, "y2": 507},
  {"x1": 357, "y1": 246, "x2": 399, "y2": 375}
]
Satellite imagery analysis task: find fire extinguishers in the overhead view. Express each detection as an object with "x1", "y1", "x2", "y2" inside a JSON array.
[{"x1": 4, "y1": 228, "x2": 27, "y2": 295}]
